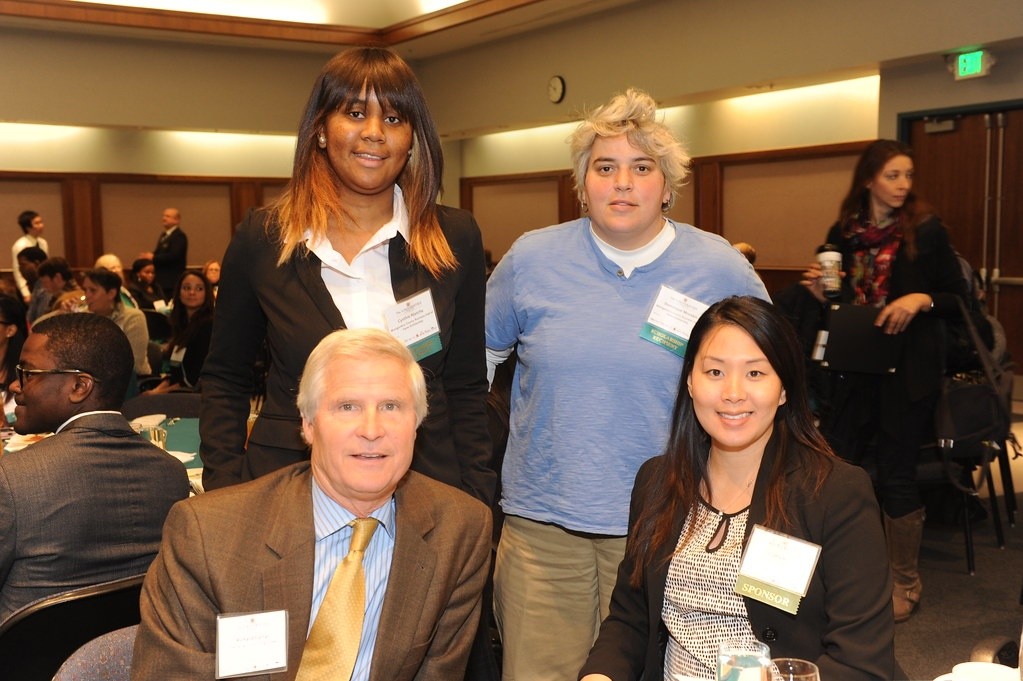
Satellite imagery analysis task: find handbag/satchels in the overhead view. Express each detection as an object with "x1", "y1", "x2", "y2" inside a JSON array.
[{"x1": 932, "y1": 293, "x2": 1012, "y2": 490}]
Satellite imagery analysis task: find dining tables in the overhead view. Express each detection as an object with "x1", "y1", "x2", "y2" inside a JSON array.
[{"x1": 3, "y1": 417, "x2": 202, "y2": 484}]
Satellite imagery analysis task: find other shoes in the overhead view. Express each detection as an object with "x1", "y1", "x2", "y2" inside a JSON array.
[{"x1": 916, "y1": 458, "x2": 989, "y2": 526}]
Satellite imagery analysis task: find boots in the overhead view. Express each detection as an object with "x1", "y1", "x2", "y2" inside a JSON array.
[{"x1": 883, "y1": 505, "x2": 926, "y2": 621}]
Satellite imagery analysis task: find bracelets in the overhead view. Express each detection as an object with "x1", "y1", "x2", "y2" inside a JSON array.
[{"x1": 930, "y1": 301, "x2": 933, "y2": 308}]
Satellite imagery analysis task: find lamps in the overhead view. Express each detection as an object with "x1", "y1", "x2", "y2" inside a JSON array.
[{"x1": 947, "y1": 50, "x2": 997, "y2": 81}]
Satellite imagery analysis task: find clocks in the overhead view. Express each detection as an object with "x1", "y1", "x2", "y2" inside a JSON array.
[{"x1": 547, "y1": 75, "x2": 565, "y2": 104}]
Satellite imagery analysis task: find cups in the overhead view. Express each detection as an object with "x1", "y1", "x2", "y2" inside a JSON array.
[
  {"x1": 138, "y1": 426, "x2": 167, "y2": 451},
  {"x1": 71, "y1": 294, "x2": 92, "y2": 313},
  {"x1": 716, "y1": 639, "x2": 782, "y2": 681},
  {"x1": 814, "y1": 243, "x2": 844, "y2": 299},
  {"x1": 769, "y1": 657, "x2": 821, "y2": 681}
]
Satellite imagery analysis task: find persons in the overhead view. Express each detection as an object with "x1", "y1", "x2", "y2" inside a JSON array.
[
  {"x1": 0, "y1": 312, "x2": 189, "y2": 628},
  {"x1": 732, "y1": 242, "x2": 756, "y2": 265},
  {"x1": 578, "y1": 296, "x2": 908, "y2": 680},
  {"x1": 799, "y1": 141, "x2": 1005, "y2": 621},
  {"x1": 0, "y1": 207, "x2": 221, "y2": 428},
  {"x1": 128, "y1": 329, "x2": 492, "y2": 681},
  {"x1": 486, "y1": 88, "x2": 773, "y2": 681},
  {"x1": 197, "y1": 46, "x2": 492, "y2": 681}
]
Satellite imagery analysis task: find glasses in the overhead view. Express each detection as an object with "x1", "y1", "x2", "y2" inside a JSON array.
[{"x1": 16, "y1": 365, "x2": 102, "y2": 389}]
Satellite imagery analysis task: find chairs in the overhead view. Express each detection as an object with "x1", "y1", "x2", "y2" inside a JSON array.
[
  {"x1": 137, "y1": 342, "x2": 163, "y2": 395},
  {"x1": 140, "y1": 309, "x2": 172, "y2": 340},
  {"x1": 0, "y1": 573, "x2": 147, "y2": 681},
  {"x1": 922, "y1": 369, "x2": 1014, "y2": 575}
]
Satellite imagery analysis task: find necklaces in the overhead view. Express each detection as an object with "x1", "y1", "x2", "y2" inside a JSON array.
[
  {"x1": 709, "y1": 448, "x2": 755, "y2": 516},
  {"x1": 875, "y1": 219, "x2": 888, "y2": 227}
]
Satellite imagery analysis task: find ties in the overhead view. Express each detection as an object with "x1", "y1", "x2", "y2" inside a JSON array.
[{"x1": 295, "y1": 516, "x2": 378, "y2": 681}]
